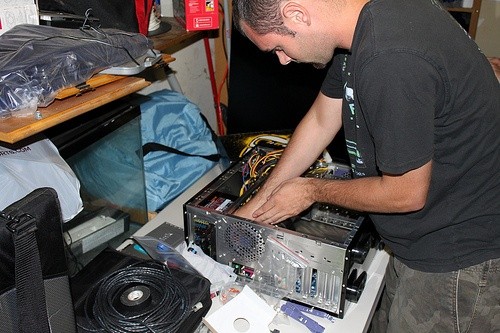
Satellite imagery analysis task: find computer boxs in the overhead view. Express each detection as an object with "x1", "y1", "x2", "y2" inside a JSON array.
[{"x1": 183, "y1": 142, "x2": 384, "y2": 320}]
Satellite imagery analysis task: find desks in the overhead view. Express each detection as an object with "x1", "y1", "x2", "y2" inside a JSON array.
[
  {"x1": 105, "y1": 157, "x2": 391, "y2": 333},
  {"x1": 140, "y1": 10, "x2": 230, "y2": 95}
]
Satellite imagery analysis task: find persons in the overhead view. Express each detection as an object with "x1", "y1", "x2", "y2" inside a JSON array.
[{"x1": 232, "y1": 0, "x2": 499, "y2": 332}]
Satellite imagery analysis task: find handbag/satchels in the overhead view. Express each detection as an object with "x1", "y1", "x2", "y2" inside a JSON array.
[
  {"x1": 1, "y1": 185, "x2": 77, "y2": 332},
  {"x1": 70, "y1": 89, "x2": 222, "y2": 211}
]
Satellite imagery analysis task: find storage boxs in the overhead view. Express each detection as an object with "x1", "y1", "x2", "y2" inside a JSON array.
[{"x1": 185, "y1": 0, "x2": 220, "y2": 32}]
